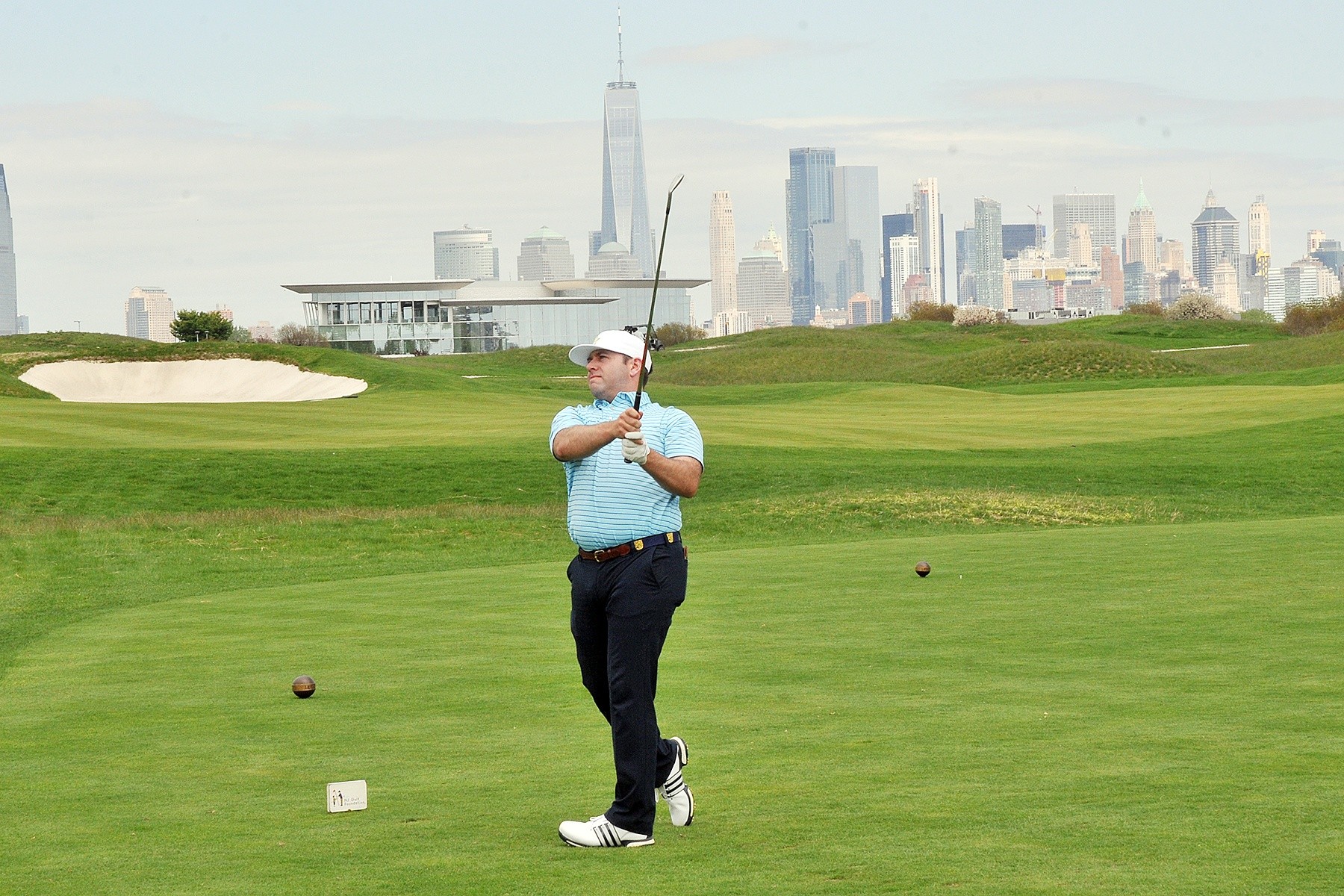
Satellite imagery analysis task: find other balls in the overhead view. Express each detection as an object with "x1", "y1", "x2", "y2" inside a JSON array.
[
  {"x1": 292, "y1": 676, "x2": 315, "y2": 698},
  {"x1": 916, "y1": 563, "x2": 931, "y2": 577}
]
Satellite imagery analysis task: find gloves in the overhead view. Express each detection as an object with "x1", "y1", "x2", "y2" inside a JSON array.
[{"x1": 620, "y1": 430, "x2": 651, "y2": 465}]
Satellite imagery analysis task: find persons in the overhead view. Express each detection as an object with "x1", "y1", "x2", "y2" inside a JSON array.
[{"x1": 549, "y1": 331, "x2": 705, "y2": 847}]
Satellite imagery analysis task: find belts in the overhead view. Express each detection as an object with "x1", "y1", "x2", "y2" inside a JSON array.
[{"x1": 579, "y1": 531, "x2": 681, "y2": 562}]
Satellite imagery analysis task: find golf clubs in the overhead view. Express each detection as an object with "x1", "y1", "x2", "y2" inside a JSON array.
[{"x1": 622, "y1": 174, "x2": 684, "y2": 463}]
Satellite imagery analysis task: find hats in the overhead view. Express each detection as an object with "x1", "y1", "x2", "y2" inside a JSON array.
[{"x1": 568, "y1": 330, "x2": 652, "y2": 375}]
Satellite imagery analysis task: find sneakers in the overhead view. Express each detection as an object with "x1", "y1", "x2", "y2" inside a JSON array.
[
  {"x1": 558, "y1": 814, "x2": 656, "y2": 849},
  {"x1": 658, "y1": 735, "x2": 694, "y2": 827}
]
